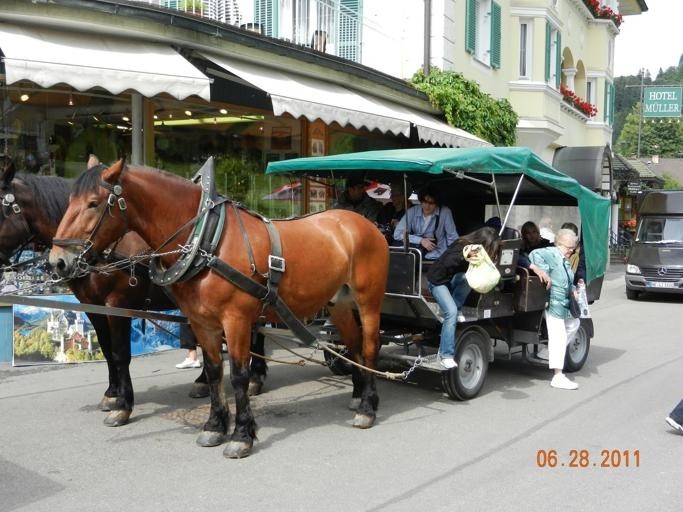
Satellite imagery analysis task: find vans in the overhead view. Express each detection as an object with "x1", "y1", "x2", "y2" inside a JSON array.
[{"x1": 0, "y1": 143, "x2": 614, "y2": 462}]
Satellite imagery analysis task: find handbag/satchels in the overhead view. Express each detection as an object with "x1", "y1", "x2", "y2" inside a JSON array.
[
  {"x1": 387, "y1": 237, "x2": 437, "y2": 259},
  {"x1": 569, "y1": 293, "x2": 581, "y2": 319},
  {"x1": 462, "y1": 244, "x2": 501, "y2": 294}
]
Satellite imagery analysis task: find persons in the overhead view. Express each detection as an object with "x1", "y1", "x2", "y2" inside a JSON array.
[{"x1": 665, "y1": 398, "x2": 683, "y2": 433}]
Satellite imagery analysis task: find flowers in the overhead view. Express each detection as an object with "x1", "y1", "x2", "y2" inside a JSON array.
[
  {"x1": 559, "y1": 83, "x2": 598, "y2": 118},
  {"x1": 582, "y1": 0, "x2": 623, "y2": 28}
]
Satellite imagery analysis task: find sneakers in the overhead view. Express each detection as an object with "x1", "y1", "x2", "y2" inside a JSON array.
[
  {"x1": 551, "y1": 374, "x2": 578, "y2": 389},
  {"x1": 175, "y1": 357, "x2": 201, "y2": 368},
  {"x1": 439, "y1": 358, "x2": 458, "y2": 369},
  {"x1": 666, "y1": 416, "x2": 683, "y2": 432},
  {"x1": 455, "y1": 309, "x2": 466, "y2": 322}
]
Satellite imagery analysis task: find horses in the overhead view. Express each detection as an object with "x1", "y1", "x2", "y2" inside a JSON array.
[
  {"x1": 0, "y1": 154, "x2": 270, "y2": 428},
  {"x1": 48, "y1": 153, "x2": 390, "y2": 459}
]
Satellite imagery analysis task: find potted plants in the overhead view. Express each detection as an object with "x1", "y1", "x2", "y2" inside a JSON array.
[{"x1": 178, "y1": 1, "x2": 208, "y2": 17}]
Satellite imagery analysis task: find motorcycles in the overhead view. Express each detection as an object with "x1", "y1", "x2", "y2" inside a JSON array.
[{"x1": 620, "y1": 224, "x2": 636, "y2": 249}]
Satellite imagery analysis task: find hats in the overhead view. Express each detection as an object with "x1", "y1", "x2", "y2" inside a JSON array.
[{"x1": 345, "y1": 174, "x2": 371, "y2": 186}]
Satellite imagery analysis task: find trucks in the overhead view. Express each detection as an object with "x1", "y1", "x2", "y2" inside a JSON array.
[{"x1": 626, "y1": 189, "x2": 683, "y2": 299}]
